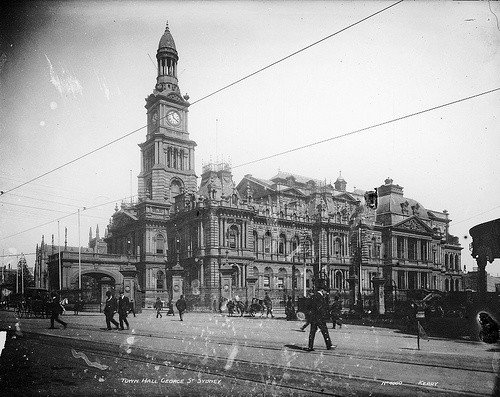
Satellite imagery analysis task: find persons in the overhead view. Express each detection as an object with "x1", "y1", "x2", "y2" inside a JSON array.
[
  {"x1": 176, "y1": 295, "x2": 187, "y2": 321},
  {"x1": 116, "y1": 292, "x2": 131, "y2": 330},
  {"x1": 308, "y1": 278, "x2": 337, "y2": 351},
  {"x1": 264, "y1": 292, "x2": 275, "y2": 318},
  {"x1": 103, "y1": 291, "x2": 119, "y2": 330},
  {"x1": 227, "y1": 300, "x2": 234, "y2": 317},
  {"x1": 331, "y1": 295, "x2": 344, "y2": 329},
  {"x1": 299, "y1": 289, "x2": 314, "y2": 332},
  {"x1": 166, "y1": 298, "x2": 175, "y2": 316},
  {"x1": 128, "y1": 300, "x2": 136, "y2": 317},
  {"x1": 154, "y1": 297, "x2": 163, "y2": 318},
  {"x1": 73, "y1": 299, "x2": 78, "y2": 316},
  {"x1": 47, "y1": 294, "x2": 67, "y2": 329},
  {"x1": 286, "y1": 296, "x2": 297, "y2": 321}
]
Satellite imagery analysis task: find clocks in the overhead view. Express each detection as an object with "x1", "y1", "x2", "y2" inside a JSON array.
[
  {"x1": 166, "y1": 109, "x2": 181, "y2": 126},
  {"x1": 151, "y1": 111, "x2": 158, "y2": 126}
]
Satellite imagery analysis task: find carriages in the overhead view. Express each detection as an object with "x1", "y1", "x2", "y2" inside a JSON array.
[
  {"x1": 233, "y1": 298, "x2": 265, "y2": 317},
  {"x1": 13, "y1": 288, "x2": 69, "y2": 319}
]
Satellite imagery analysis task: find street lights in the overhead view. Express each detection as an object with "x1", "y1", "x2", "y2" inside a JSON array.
[
  {"x1": 176, "y1": 239, "x2": 179, "y2": 265},
  {"x1": 194, "y1": 257, "x2": 203, "y2": 286},
  {"x1": 127, "y1": 238, "x2": 131, "y2": 265}
]
